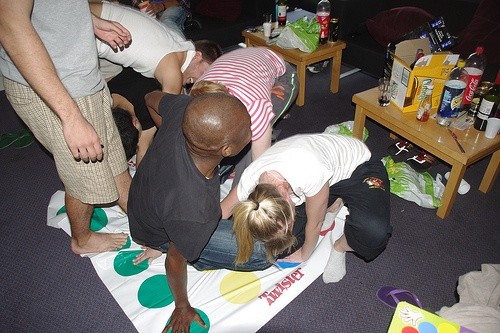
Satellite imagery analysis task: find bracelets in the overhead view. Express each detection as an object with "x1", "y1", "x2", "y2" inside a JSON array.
[{"x1": 161, "y1": 1, "x2": 166, "y2": 11}]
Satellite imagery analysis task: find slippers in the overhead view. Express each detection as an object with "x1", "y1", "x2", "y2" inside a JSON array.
[
  {"x1": 376, "y1": 284, "x2": 422, "y2": 311},
  {"x1": 0, "y1": 130, "x2": 26, "y2": 150},
  {"x1": 13, "y1": 137, "x2": 36, "y2": 149}
]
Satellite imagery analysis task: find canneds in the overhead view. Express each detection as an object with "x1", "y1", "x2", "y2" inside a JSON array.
[{"x1": 468, "y1": 82, "x2": 493, "y2": 117}]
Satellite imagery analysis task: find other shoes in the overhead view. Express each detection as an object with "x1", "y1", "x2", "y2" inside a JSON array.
[{"x1": 387, "y1": 139, "x2": 440, "y2": 172}]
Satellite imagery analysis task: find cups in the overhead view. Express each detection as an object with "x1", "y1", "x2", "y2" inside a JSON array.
[
  {"x1": 485, "y1": 118, "x2": 500, "y2": 139},
  {"x1": 378, "y1": 77, "x2": 392, "y2": 106},
  {"x1": 263, "y1": 24, "x2": 271, "y2": 36},
  {"x1": 263, "y1": 13, "x2": 272, "y2": 22}
]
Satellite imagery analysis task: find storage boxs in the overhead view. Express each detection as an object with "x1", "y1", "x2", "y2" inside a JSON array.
[{"x1": 385, "y1": 38, "x2": 461, "y2": 114}]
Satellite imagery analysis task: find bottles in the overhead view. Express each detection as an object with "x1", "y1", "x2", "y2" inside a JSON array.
[
  {"x1": 278, "y1": 0, "x2": 287, "y2": 27},
  {"x1": 435, "y1": 60, "x2": 468, "y2": 127},
  {"x1": 415, "y1": 84, "x2": 434, "y2": 122},
  {"x1": 474, "y1": 73, "x2": 500, "y2": 131},
  {"x1": 445, "y1": 171, "x2": 470, "y2": 195},
  {"x1": 316, "y1": 0, "x2": 330, "y2": 44},
  {"x1": 459, "y1": 47, "x2": 487, "y2": 111},
  {"x1": 410, "y1": 49, "x2": 424, "y2": 69}
]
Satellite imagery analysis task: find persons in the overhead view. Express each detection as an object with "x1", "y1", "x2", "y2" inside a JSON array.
[
  {"x1": 0, "y1": 0, "x2": 133, "y2": 256},
  {"x1": 90, "y1": 0, "x2": 394, "y2": 333}
]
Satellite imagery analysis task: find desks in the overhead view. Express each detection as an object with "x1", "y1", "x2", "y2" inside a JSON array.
[
  {"x1": 241, "y1": 24, "x2": 346, "y2": 107},
  {"x1": 350, "y1": 82, "x2": 500, "y2": 218}
]
